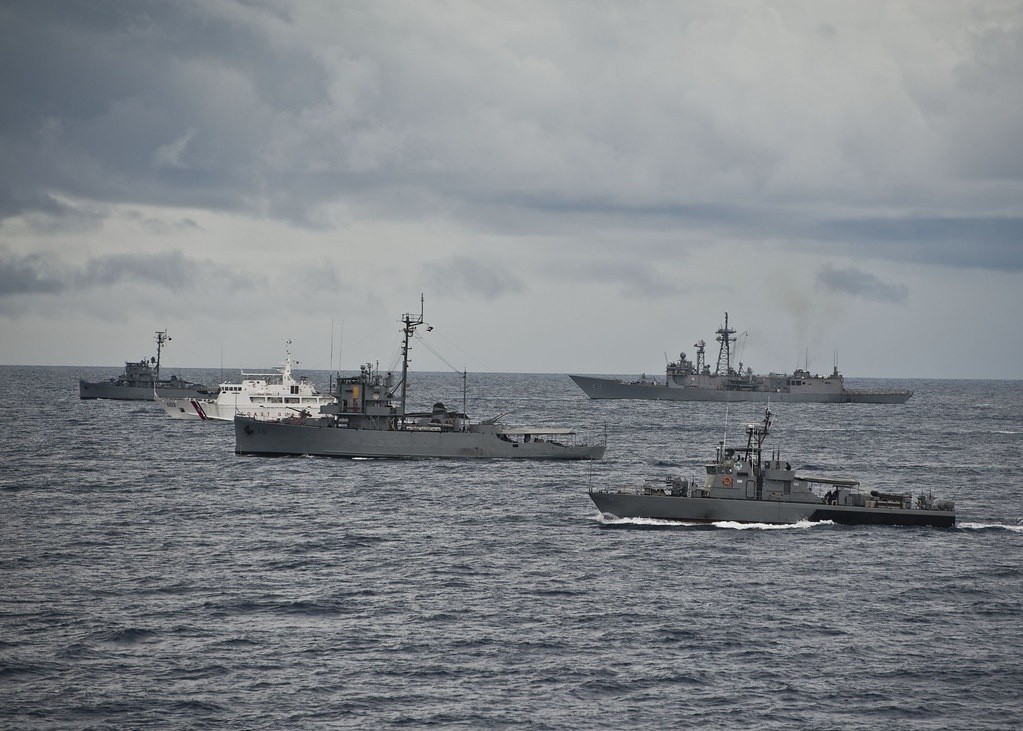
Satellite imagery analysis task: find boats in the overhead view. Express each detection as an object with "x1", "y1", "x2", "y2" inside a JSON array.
[
  {"x1": 588, "y1": 402, "x2": 960, "y2": 535},
  {"x1": 154, "y1": 341, "x2": 336, "y2": 424},
  {"x1": 566, "y1": 309, "x2": 913, "y2": 403},
  {"x1": 234, "y1": 293, "x2": 609, "y2": 464},
  {"x1": 78, "y1": 332, "x2": 220, "y2": 402}
]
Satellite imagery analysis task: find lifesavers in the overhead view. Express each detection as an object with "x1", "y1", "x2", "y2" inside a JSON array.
[{"x1": 723, "y1": 477, "x2": 732, "y2": 485}]
